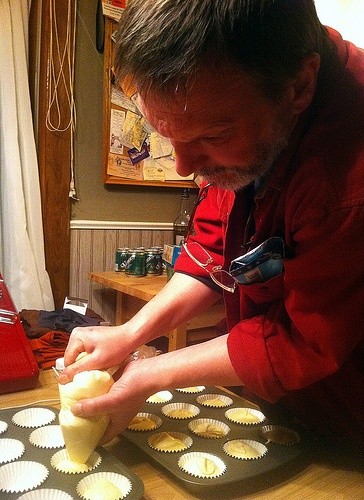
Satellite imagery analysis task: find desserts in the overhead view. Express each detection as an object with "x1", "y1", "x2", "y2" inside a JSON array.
[
  {"x1": 49, "y1": 446, "x2": 133, "y2": 500},
  {"x1": 125, "y1": 386, "x2": 301, "y2": 479}
]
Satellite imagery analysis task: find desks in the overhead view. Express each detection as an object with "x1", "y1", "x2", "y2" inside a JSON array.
[{"x1": 0, "y1": 345, "x2": 364, "y2": 500}]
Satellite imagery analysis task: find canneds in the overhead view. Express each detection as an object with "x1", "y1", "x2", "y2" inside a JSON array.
[{"x1": 114, "y1": 246, "x2": 168, "y2": 277}]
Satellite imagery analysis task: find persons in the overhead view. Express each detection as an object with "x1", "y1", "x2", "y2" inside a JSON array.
[{"x1": 57, "y1": 0, "x2": 364, "y2": 446}]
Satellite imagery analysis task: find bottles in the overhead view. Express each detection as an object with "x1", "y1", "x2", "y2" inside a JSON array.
[{"x1": 172, "y1": 188, "x2": 192, "y2": 246}]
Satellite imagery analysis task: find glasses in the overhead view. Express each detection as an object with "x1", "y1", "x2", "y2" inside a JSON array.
[{"x1": 181, "y1": 181, "x2": 253, "y2": 294}]
